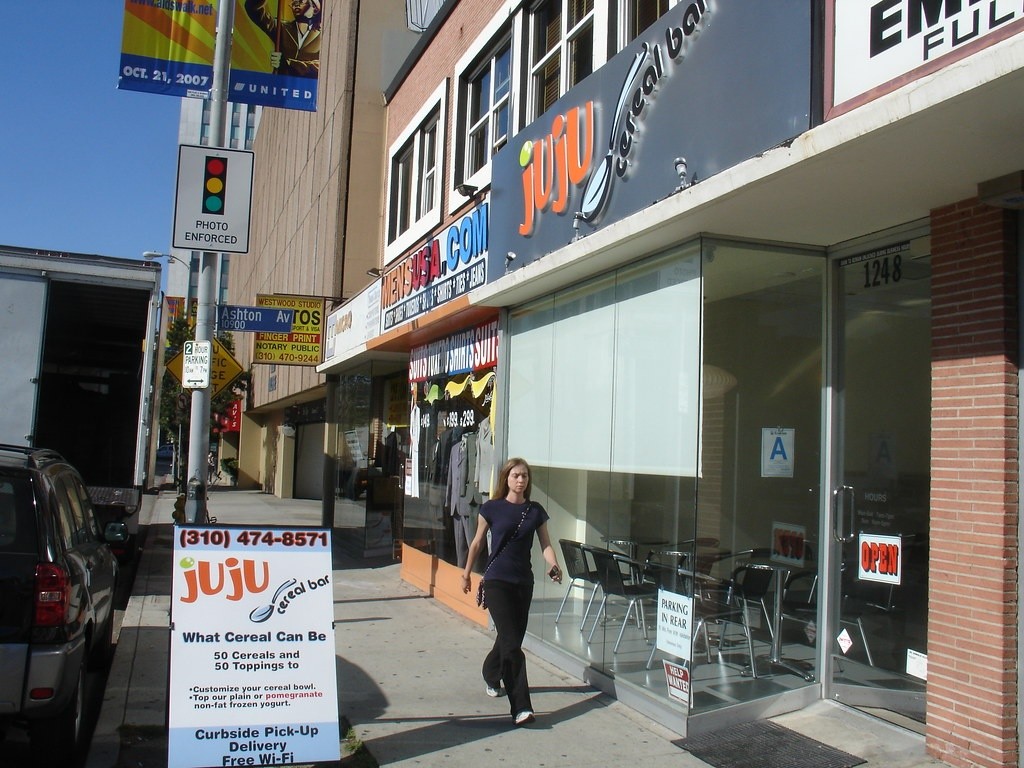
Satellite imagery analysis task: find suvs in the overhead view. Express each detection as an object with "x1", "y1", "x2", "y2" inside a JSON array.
[{"x1": 0, "y1": 444, "x2": 135, "y2": 762}]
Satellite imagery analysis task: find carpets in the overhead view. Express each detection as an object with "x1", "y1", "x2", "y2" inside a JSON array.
[{"x1": 671, "y1": 720, "x2": 868, "y2": 768}]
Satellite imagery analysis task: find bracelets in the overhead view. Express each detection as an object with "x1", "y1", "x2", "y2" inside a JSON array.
[{"x1": 462, "y1": 575, "x2": 470, "y2": 580}]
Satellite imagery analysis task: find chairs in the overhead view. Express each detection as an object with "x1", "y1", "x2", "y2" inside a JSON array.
[
  {"x1": 0, "y1": 493, "x2": 17, "y2": 548},
  {"x1": 559, "y1": 538, "x2": 873, "y2": 679},
  {"x1": 866, "y1": 532, "x2": 929, "y2": 612}
]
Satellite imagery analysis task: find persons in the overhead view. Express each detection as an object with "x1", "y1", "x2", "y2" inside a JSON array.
[
  {"x1": 207, "y1": 451, "x2": 215, "y2": 484},
  {"x1": 462, "y1": 458, "x2": 563, "y2": 724},
  {"x1": 423, "y1": 407, "x2": 493, "y2": 573}
]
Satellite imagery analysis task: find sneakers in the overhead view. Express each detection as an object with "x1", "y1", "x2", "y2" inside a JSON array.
[
  {"x1": 512, "y1": 710, "x2": 536, "y2": 725},
  {"x1": 481, "y1": 671, "x2": 502, "y2": 697}
]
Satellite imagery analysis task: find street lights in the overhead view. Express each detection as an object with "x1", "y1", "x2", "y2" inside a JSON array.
[{"x1": 144, "y1": 251, "x2": 191, "y2": 492}]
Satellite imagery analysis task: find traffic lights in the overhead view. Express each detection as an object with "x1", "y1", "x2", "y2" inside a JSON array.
[{"x1": 172, "y1": 143, "x2": 256, "y2": 258}]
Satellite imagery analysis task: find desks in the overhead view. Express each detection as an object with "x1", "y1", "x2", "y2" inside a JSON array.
[
  {"x1": 601, "y1": 536, "x2": 670, "y2": 624},
  {"x1": 735, "y1": 556, "x2": 848, "y2": 681},
  {"x1": 652, "y1": 545, "x2": 733, "y2": 653}
]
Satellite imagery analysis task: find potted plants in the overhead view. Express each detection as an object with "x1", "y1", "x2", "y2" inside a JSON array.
[{"x1": 220, "y1": 457, "x2": 238, "y2": 486}]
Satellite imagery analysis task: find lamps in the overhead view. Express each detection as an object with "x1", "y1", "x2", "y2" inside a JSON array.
[
  {"x1": 456, "y1": 184, "x2": 478, "y2": 199},
  {"x1": 671, "y1": 158, "x2": 694, "y2": 192},
  {"x1": 504, "y1": 252, "x2": 516, "y2": 275},
  {"x1": 568, "y1": 212, "x2": 585, "y2": 243},
  {"x1": 366, "y1": 268, "x2": 383, "y2": 278}
]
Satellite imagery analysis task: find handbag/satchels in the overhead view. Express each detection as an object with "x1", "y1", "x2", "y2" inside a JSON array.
[{"x1": 476, "y1": 577, "x2": 488, "y2": 610}]
